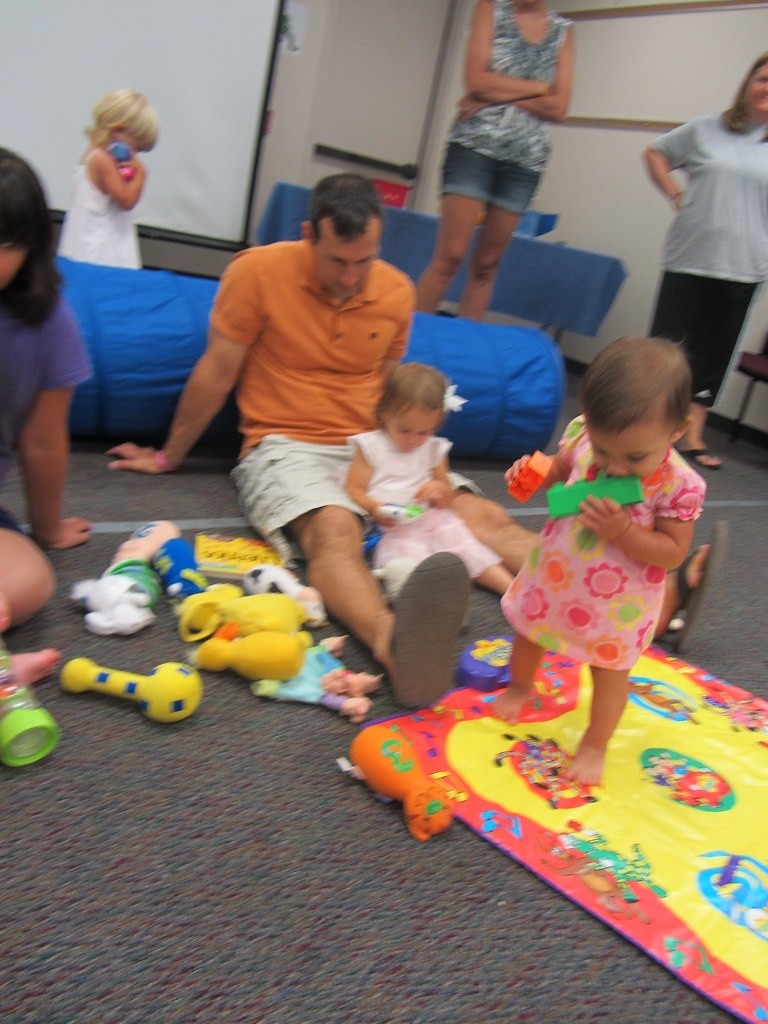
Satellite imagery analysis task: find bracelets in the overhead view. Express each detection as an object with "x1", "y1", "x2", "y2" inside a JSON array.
[
  {"x1": 152, "y1": 450, "x2": 176, "y2": 474},
  {"x1": 670, "y1": 191, "x2": 683, "y2": 203}
]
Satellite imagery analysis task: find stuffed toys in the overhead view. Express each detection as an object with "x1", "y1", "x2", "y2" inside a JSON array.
[{"x1": 68, "y1": 519, "x2": 385, "y2": 725}]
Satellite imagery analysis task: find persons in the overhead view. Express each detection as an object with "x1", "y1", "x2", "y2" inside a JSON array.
[
  {"x1": 504, "y1": 338, "x2": 706, "y2": 785},
  {"x1": 344, "y1": 360, "x2": 513, "y2": 611},
  {"x1": 105, "y1": 172, "x2": 728, "y2": 707},
  {"x1": 0, "y1": 148, "x2": 91, "y2": 687},
  {"x1": 413, "y1": 1, "x2": 579, "y2": 320},
  {"x1": 56, "y1": 91, "x2": 159, "y2": 270},
  {"x1": 643, "y1": 53, "x2": 768, "y2": 466}
]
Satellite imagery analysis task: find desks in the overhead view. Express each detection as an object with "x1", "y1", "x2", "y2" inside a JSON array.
[{"x1": 257, "y1": 182, "x2": 627, "y2": 345}]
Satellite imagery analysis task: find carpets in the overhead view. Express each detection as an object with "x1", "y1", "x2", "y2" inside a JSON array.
[{"x1": 363, "y1": 640, "x2": 768, "y2": 1023}]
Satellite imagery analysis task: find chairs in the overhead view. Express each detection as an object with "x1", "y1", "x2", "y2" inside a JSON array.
[{"x1": 729, "y1": 329, "x2": 768, "y2": 443}]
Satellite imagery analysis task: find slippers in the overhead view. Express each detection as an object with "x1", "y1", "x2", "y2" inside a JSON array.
[
  {"x1": 673, "y1": 518, "x2": 725, "y2": 655},
  {"x1": 388, "y1": 551, "x2": 470, "y2": 706},
  {"x1": 676, "y1": 443, "x2": 720, "y2": 469}
]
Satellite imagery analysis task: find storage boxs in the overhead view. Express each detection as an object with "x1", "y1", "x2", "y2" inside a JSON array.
[{"x1": 367, "y1": 178, "x2": 414, "y2": 207}]
